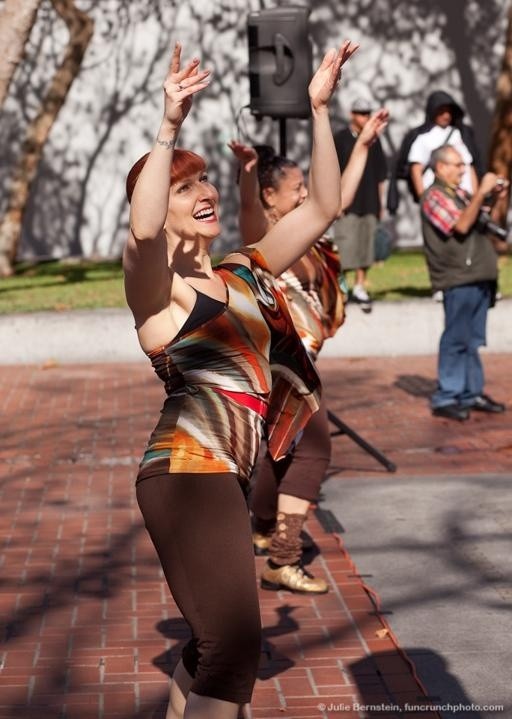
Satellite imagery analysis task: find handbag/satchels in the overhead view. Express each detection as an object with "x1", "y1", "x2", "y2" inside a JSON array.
[{"x1": 374, "y1": 227, "x2": 390, "y2": 259}]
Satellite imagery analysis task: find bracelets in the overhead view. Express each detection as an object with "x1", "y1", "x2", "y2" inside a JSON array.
[{"x1": 154, "y1": 134, "x2": 177, "y2": 150}]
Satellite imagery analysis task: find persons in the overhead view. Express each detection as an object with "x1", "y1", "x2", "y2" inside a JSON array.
[
  {"x1": 404, "y1": 88, "x2": 502, "y2": 305},
  {"x1": 333, "y1": 96, "x2": 391, "y2": 305},
  {"x1": 121, "y1": 39, "x2": 362, "y2": 718},
  {"x1": 419, "y1": 144, "x2": 510, "y2": 421},
  {"x1": 225, "y1": 106, "x2": 395, "y2": 593}
]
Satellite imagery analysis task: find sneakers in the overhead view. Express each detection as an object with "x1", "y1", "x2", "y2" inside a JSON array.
[
  {"x1": 432, "y1": 291, "x2": 443, "y2": 301},
  {"x1": 353, "y1": 285, "x2": 370, "y2": 301}
]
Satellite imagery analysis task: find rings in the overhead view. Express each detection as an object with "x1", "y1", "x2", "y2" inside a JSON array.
[
  {"x1": 337, "y1": 66, "x2": 344, "y2": 80},
  {"x1": 177, "y1": 81, "x2": 183, "y2": 91}
]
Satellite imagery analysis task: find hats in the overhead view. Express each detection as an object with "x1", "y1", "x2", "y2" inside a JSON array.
[{"x1": 352, "y1": 98, "x2": 371, "y2": 111}]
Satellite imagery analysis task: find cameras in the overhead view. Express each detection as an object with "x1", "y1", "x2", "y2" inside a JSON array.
[{"x1": 477, "y1": 211, "x2": 508, "y2": 241}]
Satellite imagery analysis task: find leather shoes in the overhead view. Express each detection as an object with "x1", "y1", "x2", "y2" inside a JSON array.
[
  {"x1": 253, "y1": 533, "x2": 274, "y2": 556},
  {"x1": 470, "y1": 395, "x2": 503, "y2": 412},
  {"x1": 432, "y1": 404, "x2": 469, "y2": 419},
  {"x1": 261, "y1": 563, "x2": 327, "y2": 595}
]
